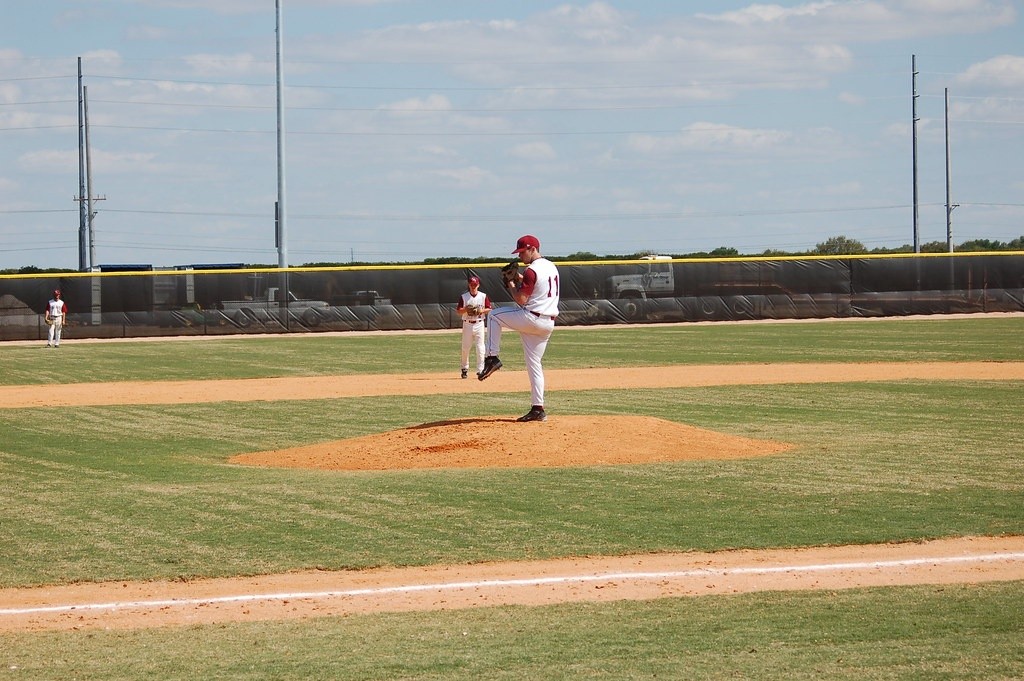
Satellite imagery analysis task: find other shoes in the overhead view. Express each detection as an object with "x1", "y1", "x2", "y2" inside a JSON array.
[
  {"x1": 45, "y1": 344, "x2": 51, "y2": 348},
  {"x1": 55, "y1": 345, "x2": 59, "y2": 348}
]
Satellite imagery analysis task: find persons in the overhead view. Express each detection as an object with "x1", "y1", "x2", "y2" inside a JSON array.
[
  {"x1": 478, "y1": 235, "x2": 560, "y2": 422},
  {"x1": 45, "y1": 290, "x2": 67, "y2": 348},
  {"x1": 456, "y1": 276, "x2": 492, "y2": 381}
]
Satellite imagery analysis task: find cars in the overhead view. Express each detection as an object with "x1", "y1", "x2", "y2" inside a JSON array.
[{"x1": 167, "y1": 301, "x2": 226, "y2": 327}]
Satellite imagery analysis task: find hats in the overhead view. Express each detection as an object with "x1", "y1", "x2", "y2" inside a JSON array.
[
  {"x1": 511, "y1": 235, "x2": 539, "y2": 254},
  {"x1": 54, "y1": 290, "x2": 60, "y2": 295},
  {"x1": 469, "y1": 277, "x2": 479, "y2": 285}
]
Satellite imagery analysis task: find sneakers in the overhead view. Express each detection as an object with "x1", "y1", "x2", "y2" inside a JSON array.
[
  {"x1": 461, "y1": 369, "x2": 468, "y2": 379},
  {"x1": 517, "y1": 409, "x2": 547, "y2": 422},
  {"x1": 478, "y1": 356, "x2": 502, "y2": 381}
]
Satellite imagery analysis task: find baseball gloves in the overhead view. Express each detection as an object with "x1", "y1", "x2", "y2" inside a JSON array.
[
  {"x1": 45, "y1": 319, "x2": 53, "y2": 325},
  {"x1": 467, "y1": 304, "x2": 482, "y2": 316},
  {"x1": 502, "y1": 260, "x2": 519, "y2": 283}
]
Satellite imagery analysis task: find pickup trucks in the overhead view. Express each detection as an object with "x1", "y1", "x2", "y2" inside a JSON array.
[{"x1": 218, "y1": 288, "x2": 332, "y2": 329}]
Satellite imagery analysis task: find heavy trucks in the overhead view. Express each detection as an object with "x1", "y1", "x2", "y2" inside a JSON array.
[{"x1": 603, "y1": 254, "x2": 794, "y2": 323}]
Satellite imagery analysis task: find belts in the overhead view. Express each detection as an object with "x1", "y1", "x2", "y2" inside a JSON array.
[
  {"x1": 530, "y1": 311, "x2": 556, "y2": 320},
  {"x1": 464, "y1": 320, "x2": 481, "y2": 323}
]
